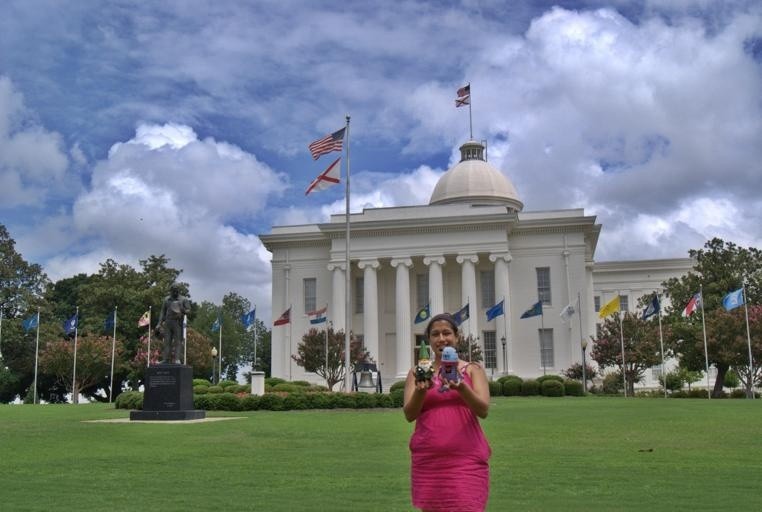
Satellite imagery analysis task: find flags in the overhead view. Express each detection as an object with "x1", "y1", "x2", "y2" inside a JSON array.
[
  {"x1": 183, "y1": 314, "x2": 188, "y2": 340},
  {"x1": 451, "y1": 302, "x2": 471, "y2": 325},
  {"x1": 457, "y1": 82, "x2": 471, "y2": 97},
  {"x1": 681, "y1": 292, "x2": 703, "y2": 319},
  {"x1": 138, "y1": 305, "x2": 152, "y2": 328},
  {"x1": 307, "y1": 305, "x2": 328, "y2": 324},
  {"x1": 64, "y1": 311, "x2": 79, "y2": 336},
  {"x1": 242, "y1": 308, "x2": 256, "y2": 328},
  {"x1": 454, "y1": 95, "x2": 472, "y2": 108},
  {"x1": 308, "y1": 126, "x2": 346, "y2": 161},
  {"x1": 599, "y1": 289, "x2": 621, "y2": 319},
  {"x1": 520, "y1": 298, "x2": 543, "y2": 319},
  {"x1": 23, "y1": 311, "x2": 39, "y2": 332},
  {"x1": 274, "y1": 305, "x2": 292, "y2": 326},
  {"x1": 103, "y1": 305, "x2": 118, "y2": 333},
  {"x1": 641, "y1": 290, "x2": 661, "y2": 320},
  {"x1": 559, "y1": 292, "x2": 580, "y2": 326},
  {"x1": 486, "y1": 299, "x2": 505, "y2": 321},
  {"x1": 211, "y1": 312, "x2": 224, "y2": 332},
  {"x1": 722, "y1": 287, "x2": 746, "y2": 311},
  {"x1": 304, "y1": 156, "x2": 343, "y2": 197},
  {"x1": 413, "y1": 302, "x2": 431, "y2": 324}
]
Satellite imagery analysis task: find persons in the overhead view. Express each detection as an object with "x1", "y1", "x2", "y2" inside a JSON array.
[
  {"x1": 413, "y1": 340, "x2": 435, "y2": 390},
  {"x1": 154, "y1": 283, "x2": 192, "y2": 364},
  {"x1": 402, "y1": 311, "x2": 493, "y2": 511},
  {"x1": 437, "y1": 343, "x2": 466, "y2": 393}
]
